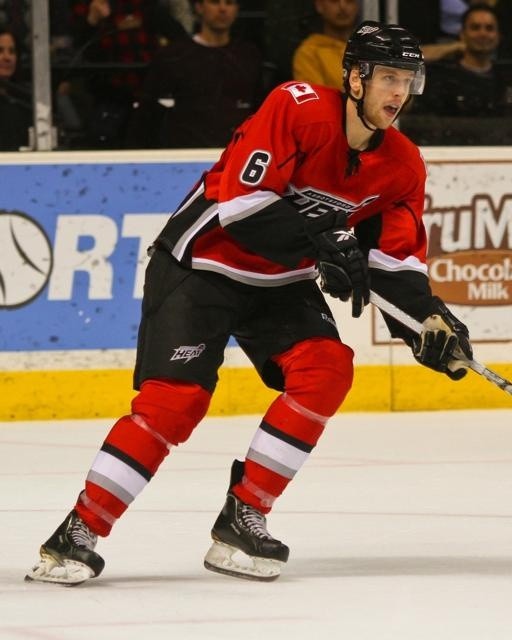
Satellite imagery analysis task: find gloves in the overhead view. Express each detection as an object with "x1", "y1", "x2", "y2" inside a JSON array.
[
  {"x1": 410, "y1": 294, "x2": 474, "y2": 381},
  {"x1": 315, "y1": 221, "x2": 372, "y2": 319}
]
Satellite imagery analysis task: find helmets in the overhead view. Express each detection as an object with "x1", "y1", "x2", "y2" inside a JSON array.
[{"x1": 341, "y1": 21, "x2": 425, "y2": 82}]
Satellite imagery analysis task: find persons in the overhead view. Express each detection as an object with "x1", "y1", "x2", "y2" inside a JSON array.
[
  {"x1": 37, "y1": 22, "x2": 473, "y2": 578},
  {"x1": 0, "y1": 0, "x2": 512, "y2": 146}
]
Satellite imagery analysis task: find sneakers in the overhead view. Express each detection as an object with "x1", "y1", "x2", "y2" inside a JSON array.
[
  {"x1": 208, "y1": 494, "x2": 290, "y2": 561},
  {"x1": 39, "y1": 508, "x2": 104, "y2": 577}
]
